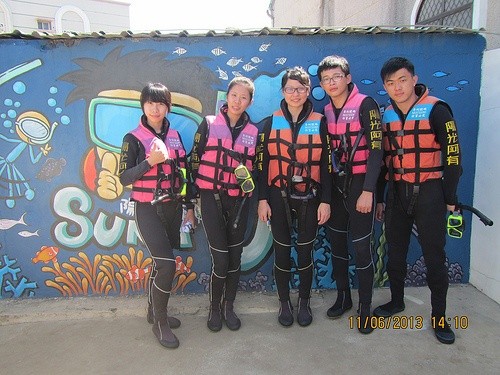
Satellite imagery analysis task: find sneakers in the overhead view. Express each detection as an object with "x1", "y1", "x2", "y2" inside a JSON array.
[
  {"x1": 432, "y1": 314, "x2": 456, "y2": 343},
  {"x1": 327, "y1": 291, "x2": 352, "y2": 318},
  {"x1": 152, "y1": 311, "x2": 179, "y2": 348},
  {"x1": 207, "y1": 302, "x2": 222, "y2": 331},
  {"x1": 297, "y1": 298, "x2": 312, "y2": 326},
  {"x1": 147, "y1": 307, "x2": 181, "y2": 329},
  {"x1": 357, "y1": 302, "x2": 375, "y2": 334},
  {"x1": 374, "y1": 300, "x2": 405, "y2": 318},
  {"x1": 278, "y1": 300, "x2": 294, "y2": 326}
]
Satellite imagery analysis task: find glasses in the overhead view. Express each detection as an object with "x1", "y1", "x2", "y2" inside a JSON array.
[
  {"x1": 171, "y1": 166, "x2": 187, "y2": 200},
  {"x1": 321, "y1": 75, "x2": 346, "y2": 83},
  {"x1": 447, "y1": 212, "x2": 463, "y2": 239},
  {"x1": 284, "y1": 87, "x2": 307, "y2": 94},
  {"x1": 234, "y1": 163, "x2": 255, "y2": 193},
  {"x1": 331, "y1": 150, "x2": 341, "y2": 173}
]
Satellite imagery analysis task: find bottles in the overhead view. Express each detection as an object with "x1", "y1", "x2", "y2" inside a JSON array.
[{"x1": 181, "y1": 222, "x2": 193, "y2": 234}]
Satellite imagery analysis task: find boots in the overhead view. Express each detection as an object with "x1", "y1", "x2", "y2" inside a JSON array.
[{"x1": 222, "y1": 300, "x2": 241, "y2": 330}]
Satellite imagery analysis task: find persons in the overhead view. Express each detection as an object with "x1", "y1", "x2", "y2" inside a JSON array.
[
  {"x1": 180, "y1": 77, "x2": 261, "y2": 333},
  {"x1": 374, "y1": 56, "x2": 463, "y2": 344},
  {"x1": 257, "y1": 66, "x2": 332, "y2": 326},
  {"x1": 317, "y1": 54, "x2": 384, "y2": 335},
  {"x1": 118, "y1": 81, "x2": 198, "y2": 349}
]
